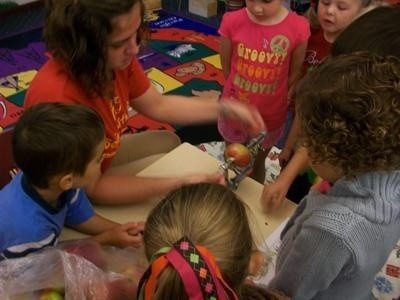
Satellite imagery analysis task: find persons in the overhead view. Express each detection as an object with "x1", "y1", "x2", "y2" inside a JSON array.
[
  {"x1": 0, "y1": 102, "x2": 145, "y2": 300},
  {"x1": 143, "y1": 183, "x2": 297, "y2": 300},
  {"x1": 25, "y1": 0, "x2": 266, "y2": 206},
  {"x1": 214, "y1": 0, "x2": 313, "y2": 174},
  {"x1": 276, "y1": 0, "x2": 367, "y2": 206},
  {"x1": 272, "y1": 52, "x2": 398, "y2": 299},
  {"x1": 261, "y1": 6, "x2": 400, "y2": 211}
]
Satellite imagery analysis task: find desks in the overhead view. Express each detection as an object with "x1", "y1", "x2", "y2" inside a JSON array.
[{"x1": 57, "y1": 141, "x2": 300, "y2": 299}]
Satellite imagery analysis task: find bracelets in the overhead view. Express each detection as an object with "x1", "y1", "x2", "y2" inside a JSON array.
[{"x1": 219, "y1": 100, "x2": 230, "y2": 119}]
